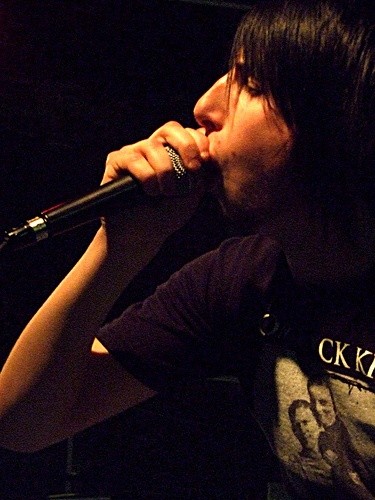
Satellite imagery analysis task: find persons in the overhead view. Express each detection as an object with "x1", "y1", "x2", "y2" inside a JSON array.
[{"x1": 1, "y1": 0, "x2": 375, "y2": 500}]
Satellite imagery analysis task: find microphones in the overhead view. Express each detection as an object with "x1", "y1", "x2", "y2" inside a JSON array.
[{"x1": 4, "y1": 146, "x2": 189, "y2": 252}]
